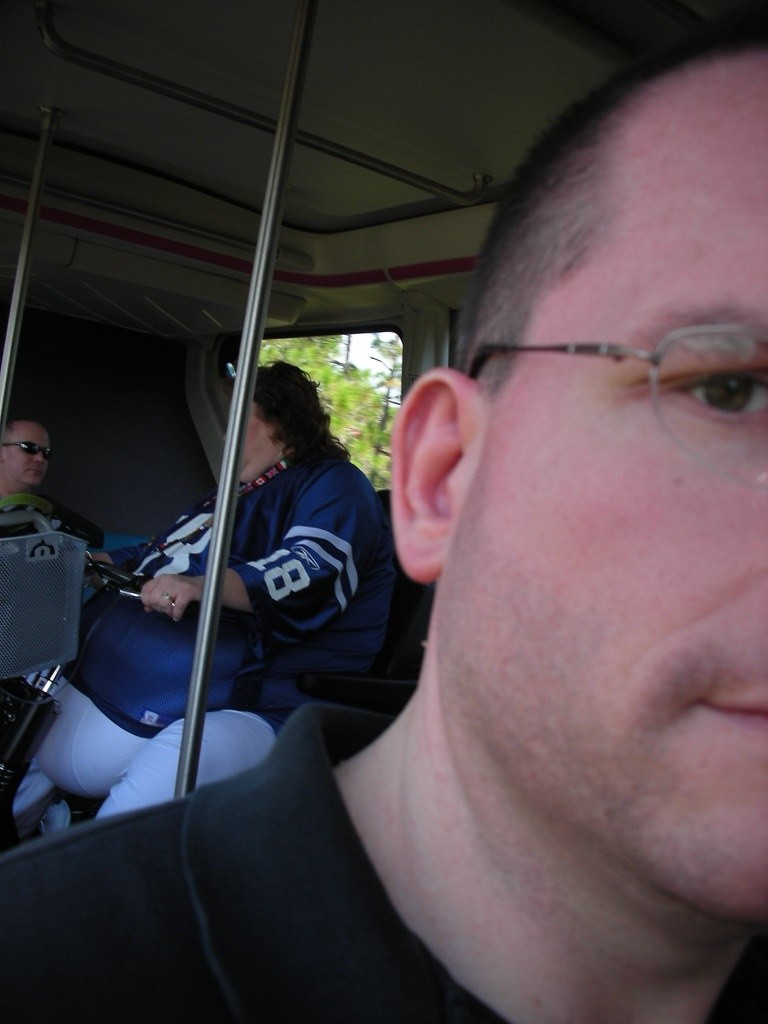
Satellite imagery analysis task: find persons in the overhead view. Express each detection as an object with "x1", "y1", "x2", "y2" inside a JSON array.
[
  {"x1": 0, "y1": 21, "x2": 768, "y2": 1024},
  {"x1": 12, "y1": 360, "x2": 397, "y2": 841},
  {"x1": 0, "y1": 418, "x2": 104, "y2": 549}
]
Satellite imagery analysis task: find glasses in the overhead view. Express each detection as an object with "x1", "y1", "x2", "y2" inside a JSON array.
[
  {"x1": 2, "y1": 441, "x2": 51, "y2": 461},
  {"x1": 468, "y1": 321, "x2": 768, "y2": 488}
]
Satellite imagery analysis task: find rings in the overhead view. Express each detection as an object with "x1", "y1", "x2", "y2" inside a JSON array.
[{"x1": 161, "y1": 593, "x2": 175, "y2": 603}]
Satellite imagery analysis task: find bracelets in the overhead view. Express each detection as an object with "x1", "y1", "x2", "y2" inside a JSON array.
[{"x1": 86, "y1": 550, "x2": 93, "y2": 559}]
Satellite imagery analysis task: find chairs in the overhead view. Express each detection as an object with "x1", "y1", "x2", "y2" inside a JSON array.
[{"x1": 293, "y1": 489, "x2": 436, "y2": 711}]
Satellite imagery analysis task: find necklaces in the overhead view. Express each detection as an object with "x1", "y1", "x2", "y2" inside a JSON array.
[{"x1": 275, "y1": 443, "x2": 294, "y2": 459}]
